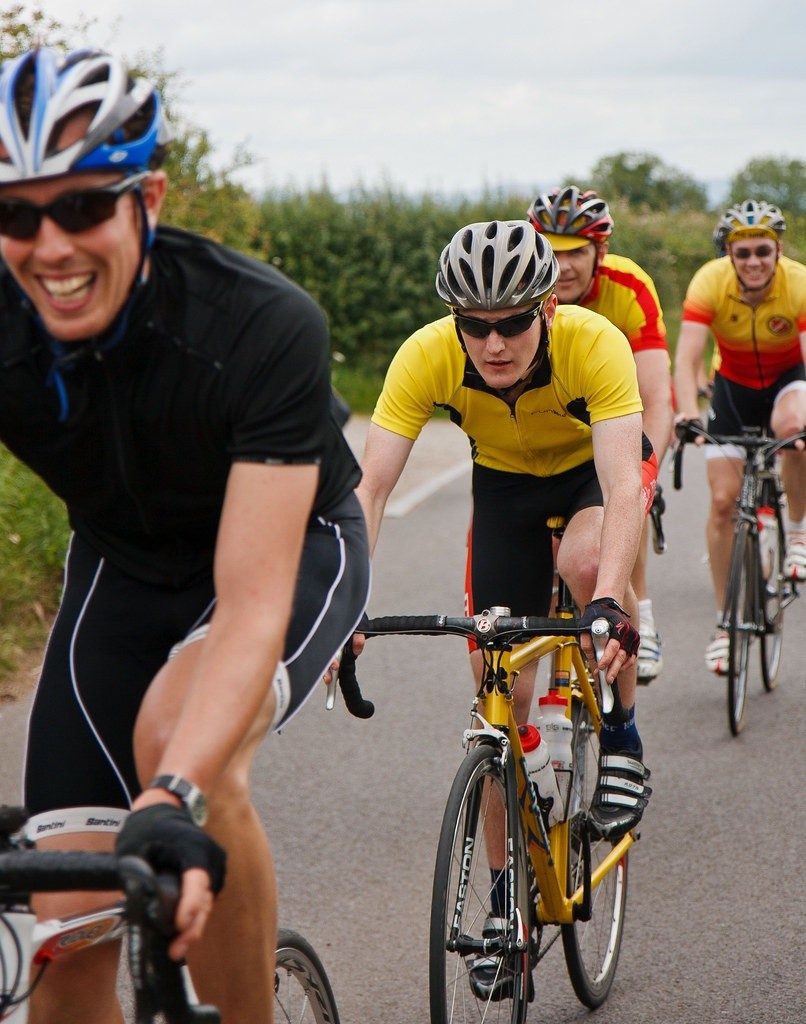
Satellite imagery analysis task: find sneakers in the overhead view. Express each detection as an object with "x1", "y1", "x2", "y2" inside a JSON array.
[
  {"x1": 706, "y1": 630, "x2": 730, "y2": 675},
  {"x1": 587, "y1": 735, "x2": 653, "y2": 839},
  {"x1": 636, "y1": 632, "x2": 662, "y2": 681},
  {"x1": 783, "y1": 530, "x2": 805, "y2": 579},
  {"x1": 468, "y1": 911, "x2": 513, "y2": 1001}
]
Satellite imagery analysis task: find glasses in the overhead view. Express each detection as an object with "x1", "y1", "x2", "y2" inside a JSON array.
[
  {"x1": 0, "y1": 170, "x2": 153, "y2": 240},
  {"x1": 450, "y1": 301, "x2": 545, "y2": 338},
  {"x1": 732, "y1": 243, "x2": 777, "y2": 258}
]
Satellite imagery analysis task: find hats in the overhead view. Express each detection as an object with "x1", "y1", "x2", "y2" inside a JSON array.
[
  {"x1": 538, "y1": 199, "x2": 589, "y2": 251},
  {"x1": 726, "y1": 214, "x2": 778, "y2": 241}
]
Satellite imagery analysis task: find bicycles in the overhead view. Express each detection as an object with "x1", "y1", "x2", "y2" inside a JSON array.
[
  {"x1": 0, "y1": 804, "x2": 342, "y2": 1024},
  {"x1": 322, "y1": 483, "x2": 668, "y2": 1024},
  {"x1": 670, "y1": 419, "x2": 806, "y2": 736}
]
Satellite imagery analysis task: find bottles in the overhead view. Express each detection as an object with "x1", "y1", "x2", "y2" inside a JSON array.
[
  {"x1": 538, "y1": 687, "x2": 576, "y2": 818},
  {"x1": 757, "y1": 506, "x2": 778, "y2": 574},
  {"x1": 757, "y1": 522, "x2": 771, "y2": 580},
  {"x1": 515, "y1": 724, "x2": 564, "y2": 832}
]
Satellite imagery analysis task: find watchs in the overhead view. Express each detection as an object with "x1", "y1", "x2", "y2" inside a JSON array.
[{"x1": 149, "y1": 773, "x2": 208, "y2": 827}]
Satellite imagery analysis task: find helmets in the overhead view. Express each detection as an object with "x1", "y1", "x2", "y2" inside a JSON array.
[
  {"x1": 526, "y1": 185, "x2": 615, "y2": 244},
  {"x1": 0, "y1": 49, "x2": 173, "y2": 183},
  {"x1": 711, "y1": 201, "x2": 786, "y2": 253},
  {"x1": 436, "y1": 220, "x2": 561, "y2": 311}
]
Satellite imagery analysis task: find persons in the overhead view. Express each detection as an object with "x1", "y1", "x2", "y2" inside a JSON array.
[
  {"x1": 671, "y1": 199, "x2": 806, "y2": 671},
  {"x1": 1, "y1": 45, "x2": 371, "y2": 1024},
  {"x1": 524, "y1": 184, "x2": 673, "y2": 678},
  {"x1": 323, "y1": 224, "x2": 643, "y2": 1001}
]
